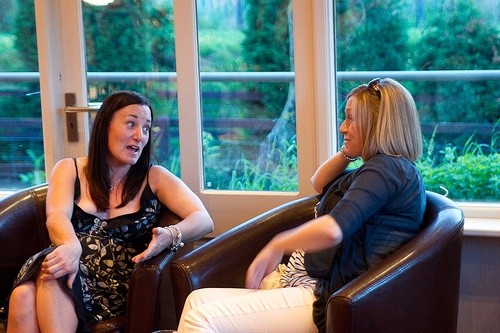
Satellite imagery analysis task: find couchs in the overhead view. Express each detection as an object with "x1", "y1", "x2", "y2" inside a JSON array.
[
  {"x1": 0, "y1": 184, "x2": 194, "y2": 333},
  {"x1": 170, "y1": 190, "x2": 464, "y2": 333}
]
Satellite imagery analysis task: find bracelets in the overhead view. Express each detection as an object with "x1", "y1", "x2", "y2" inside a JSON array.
[
  {"x1": 341, "y1": 145, "x2": 358, "y2": 163},
  {"x1": 164, "y1": 225, "x2": 184, "y2": 251}
]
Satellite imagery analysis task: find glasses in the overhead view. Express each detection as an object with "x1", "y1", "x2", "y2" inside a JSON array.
[{"x1": 368, "y1": 78, "x2": 381, "y2": 98}]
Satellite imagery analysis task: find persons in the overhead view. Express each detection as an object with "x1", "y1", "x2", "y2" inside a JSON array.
[
  {"x1": 180, "y1": 79, "x2": 425, "y2": 332},
  {"x1": 9, "y1": 91, "x2": 215, "y2": 332}
]
